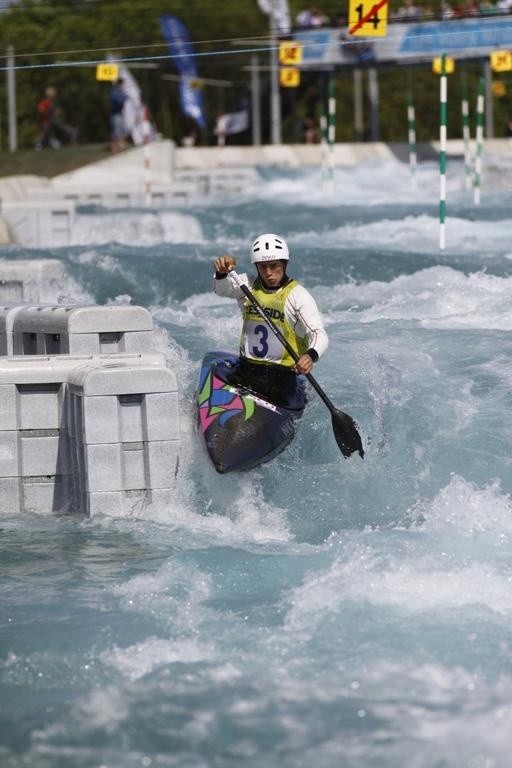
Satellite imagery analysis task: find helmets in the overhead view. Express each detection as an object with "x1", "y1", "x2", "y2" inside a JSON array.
[{"x1": 249, "y1": 234, "x2": 289, "y2": 264}]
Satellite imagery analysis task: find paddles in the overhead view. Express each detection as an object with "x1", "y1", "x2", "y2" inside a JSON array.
[{"x1": 222, "y1": 256, "x2": 367, "y2": 460}]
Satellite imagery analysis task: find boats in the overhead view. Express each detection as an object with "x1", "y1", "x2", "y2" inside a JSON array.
[{"x1": 196, "y1": 350, "x2": 309, "y2": 475}]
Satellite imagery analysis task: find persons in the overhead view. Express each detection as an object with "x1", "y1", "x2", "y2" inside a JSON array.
[
  {"x1": 214, "y1": 234, "x2": 328, "y2": 405},
  {"x1": 294, "y1": 0, "x2": 512, "y2": 28},
  {"x1": 34, "y1": 79, "x2": 324, "y2": 150}
]
